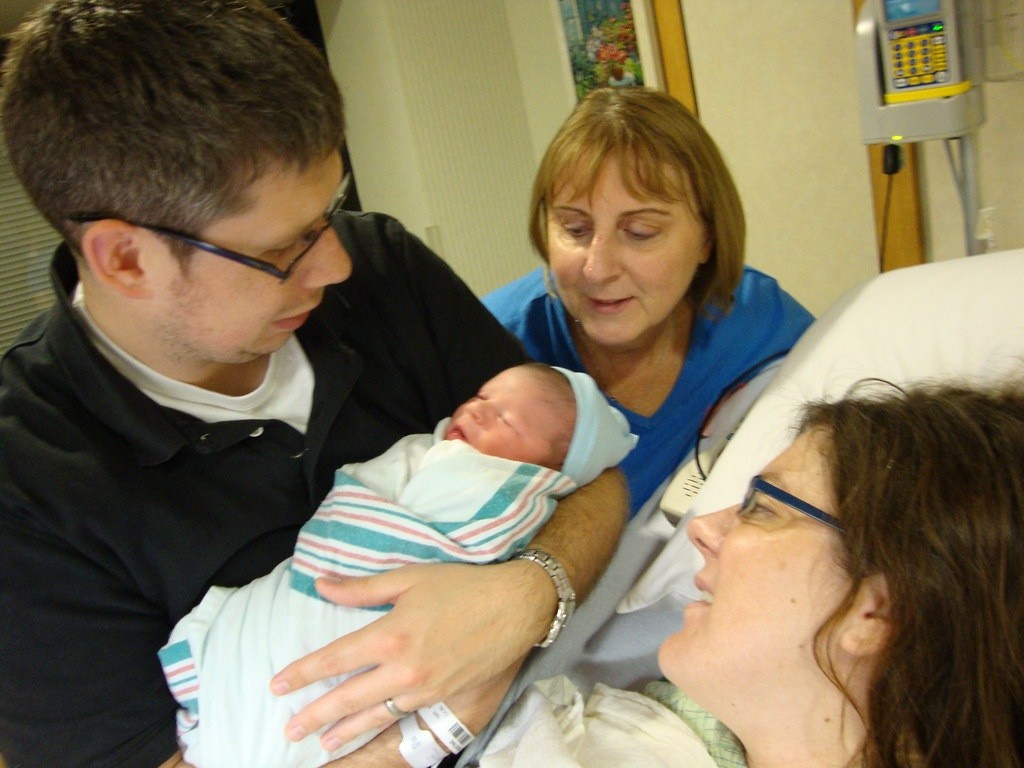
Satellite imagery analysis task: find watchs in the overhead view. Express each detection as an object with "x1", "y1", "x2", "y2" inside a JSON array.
[{"x1": 508, "y1": 549, "x2": 576, "y2": 647}]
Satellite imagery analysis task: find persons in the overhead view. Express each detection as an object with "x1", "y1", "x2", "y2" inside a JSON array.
[
  {"x1": 472, "y1": 376, "x2": 1024, "y2": 768},
  {"x1": 0, "y1": 0, "x2": 629, "y2": 768},
  {"x1": 478, "y1": 88, "x2": 816, "y2": 518},
  {"x1": 157, "y1": 363, "x2": 641, "y2": 768}
]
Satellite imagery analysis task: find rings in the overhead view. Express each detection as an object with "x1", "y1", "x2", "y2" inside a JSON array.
[{"x1": 385, "y1": 700, "x2": 411, "y2": 718}]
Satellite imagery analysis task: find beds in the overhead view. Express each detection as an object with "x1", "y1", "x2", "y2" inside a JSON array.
[{"x1": 450, "y1": 358, "x2": 697, "y2": 765}]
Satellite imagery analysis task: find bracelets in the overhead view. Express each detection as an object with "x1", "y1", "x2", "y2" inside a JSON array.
[
  {"x1": 417, "y1": 701, "x2": 476, "y2": 756},
  {"x1": 399, "y1": 714, "x2": 449, "y2": 768}
]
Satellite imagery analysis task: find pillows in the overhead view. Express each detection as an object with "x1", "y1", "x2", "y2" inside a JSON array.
[{"x1": 615, "y1": 246, "x2": 1024, "y2": 617}]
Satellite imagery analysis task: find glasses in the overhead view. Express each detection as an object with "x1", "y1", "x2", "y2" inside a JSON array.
[
  {"x1": 57, "y1": 173, "x2": 350, "y2": 282},
  {"x1": 738, "y1": 476, "x2": 848, "y2": 531}
]
